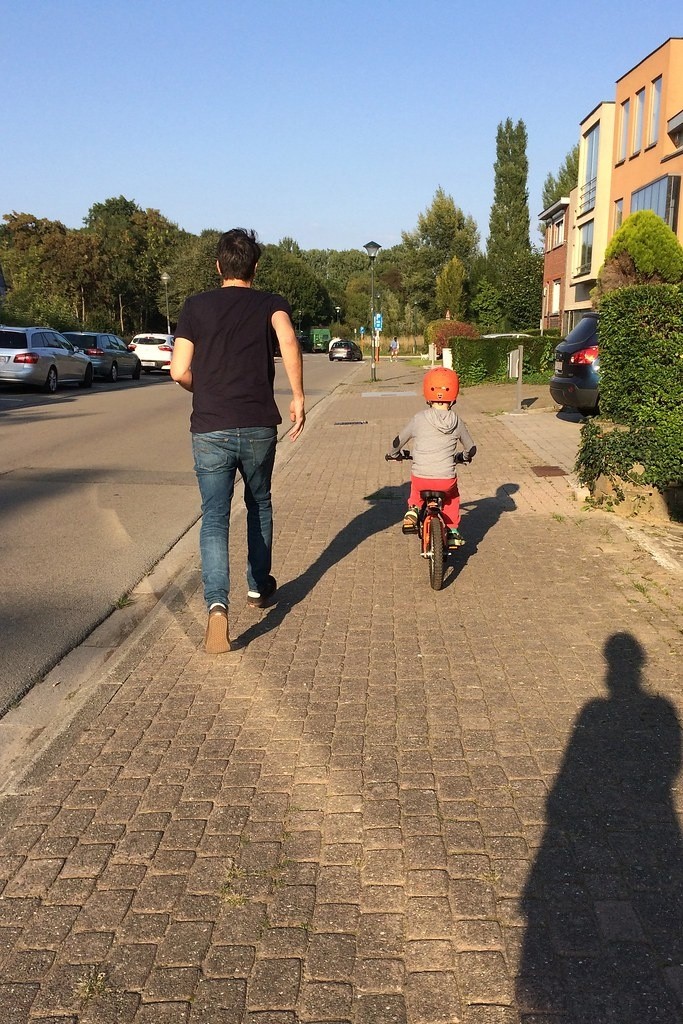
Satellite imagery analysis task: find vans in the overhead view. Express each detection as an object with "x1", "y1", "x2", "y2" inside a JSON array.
[{"x1": 308, "y1": 329, "x2": 331, "y2": 351}]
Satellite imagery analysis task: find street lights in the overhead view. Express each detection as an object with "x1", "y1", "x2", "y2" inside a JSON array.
[
  {"x1": 363, "y1": 241, "x2": 381, "y2": 381},
  {"x1": 413, "y1": 299, "x2": 419, "y2": 355},
  {"x1": 297, "y1": 309, "x2": 302, "y2": 335},
  {"x1": 161, "y1": 272, "x2": 171, "y2": 334},
  {"x1": 336, "y1": 307, "x2": 340, "y2": 338}
]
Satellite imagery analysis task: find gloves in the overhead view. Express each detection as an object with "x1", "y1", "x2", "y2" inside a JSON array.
[
  {"x1": 390, "y1": 449, "x2": 410, "y2": 461},
  {"x1": 453, "y1": 449, "x2": 473, "y2": 466}
]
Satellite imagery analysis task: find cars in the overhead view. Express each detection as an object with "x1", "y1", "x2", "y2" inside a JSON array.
[
  {"x1": 275, "y1": 336, "x2": 317, "y2": 355},
  {"x1": 129, "y1": 334, "x2": 174, "y2": 373},
  {"x1": 60, "y1": 332, "x2": 142, "y2": 381},
  {"x1": 329, "y1": 340, "x2": 363, "y2": 361},
  {"x1": 483, "y1": 333, "x2": 534, "y2": 338}
]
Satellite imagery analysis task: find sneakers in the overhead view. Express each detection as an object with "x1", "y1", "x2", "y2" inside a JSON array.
[
  {"x1": 402, "y1": 510, "x2": 418, "y2": 526},
  {"x1": 447, "y1": 532, "x2": 466, "y2": 546}
]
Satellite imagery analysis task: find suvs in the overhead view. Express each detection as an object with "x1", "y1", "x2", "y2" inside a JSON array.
[
  {"x1": 549, "y1": 312, "x2": 603, "y2": 419},
  {"x1": 0, "y1": 325, "x2": 93, "y2": 393}
]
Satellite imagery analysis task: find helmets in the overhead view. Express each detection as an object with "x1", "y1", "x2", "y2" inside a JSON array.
[{"x1": 422, "y1": 367, "x2": 460, "y2": 401}]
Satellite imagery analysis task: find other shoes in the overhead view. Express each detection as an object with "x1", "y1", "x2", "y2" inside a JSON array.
[
  {"x1": 204, "y1": 602, "x2": 232, "y2": 653},
  {"x1": 246, "y1": 575, "x2": 278, "y2": 606}
]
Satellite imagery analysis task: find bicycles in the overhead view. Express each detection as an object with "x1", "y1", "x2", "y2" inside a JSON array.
[{"x1": 390, "y1": 449, "x2": 474, "y2": 589}]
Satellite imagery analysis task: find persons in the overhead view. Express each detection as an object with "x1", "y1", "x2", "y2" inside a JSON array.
[
  {"x1": 390, "y1": 337, "x2": 399, "y2": 362},
  {"x1": 170, "y1": 229, "x2": 306, "y2": 654},
  {"x1": 391, "y1": 367, "x2": 476, "y2": 546}
]
